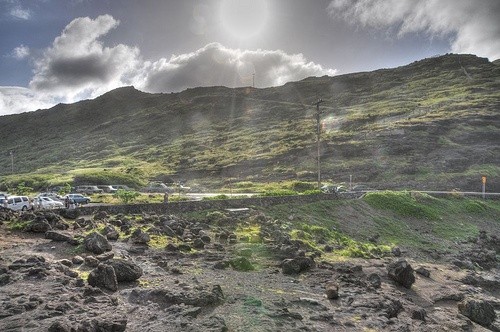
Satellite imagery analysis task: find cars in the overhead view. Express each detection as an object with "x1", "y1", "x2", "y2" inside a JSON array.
[
  {"x1": 352, "y1": 185, "x2": 367, "y2": 191},
  {"x1": 320, "y1": 185, "x2": 337, "y2": 193},
  {"x1": 147, "y1": 182, "x2": 169, "y2": 194},
  {"x1": 0, "y1": 180, "x2": 132, "y2": 212},
  {"x1": 337, "y1": 186, "x2": 347, "y2": 192}
]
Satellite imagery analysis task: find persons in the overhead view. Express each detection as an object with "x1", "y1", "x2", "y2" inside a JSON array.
[{"x1": 65, "y1": 196, "x2": 69, "y2": 208}]
